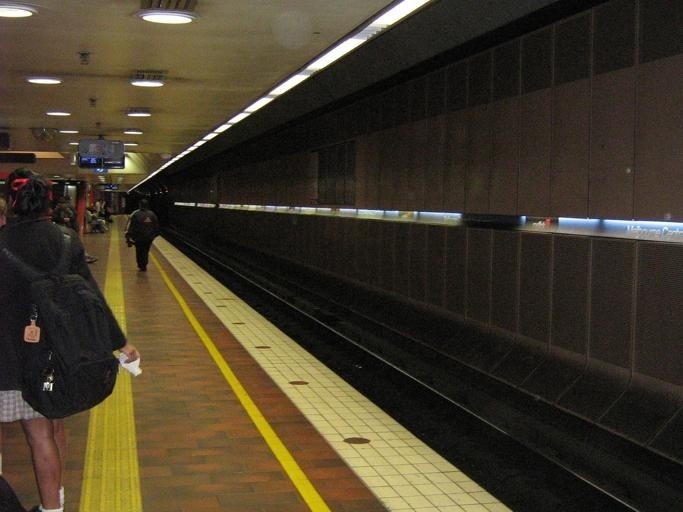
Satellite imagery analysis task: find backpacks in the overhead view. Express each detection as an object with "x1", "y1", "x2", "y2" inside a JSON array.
[{"x1": 16, "y1": 271, "x2": 120, "y2": 418}]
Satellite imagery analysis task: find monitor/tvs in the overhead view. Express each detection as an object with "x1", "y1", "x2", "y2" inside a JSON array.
[
  {"x1": 103, "y1": 184, "x2": 119, "y2": 191},
  {"x1": 77, "y1": 140, "x2": 125, "y2": 173}
]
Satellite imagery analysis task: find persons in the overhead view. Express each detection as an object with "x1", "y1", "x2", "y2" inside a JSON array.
[
  {"x1": 42, "y1": 194, "x2": 112, "y2": 236},
  {"x1": 124, "y1": 198, "x2": 160, "y2": 271},
  {"x1": 0, "y1": 166, "x2": 141, "y2": 511}
]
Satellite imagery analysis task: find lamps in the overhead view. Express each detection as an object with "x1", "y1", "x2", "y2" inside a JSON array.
[
  {"x1": 125, "y1": 108, "x2": 151, "y2": 118},
  {"x1": 130, "y1": 1, "x2": 200, "y2": 25},
  {"x1": 123, "y1": 141, "x2": 139, "y2": 146},
  {"x1": 122, "y1": 128, "x2": 143, "y2": 134},
  {"x1": 126, "y1": 70, "x2": 169, "y2": 88}
]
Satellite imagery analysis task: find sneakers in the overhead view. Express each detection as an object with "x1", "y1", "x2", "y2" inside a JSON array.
[{"x1": 84, "y1": 253, "x2": 98, "y2": 264}]
